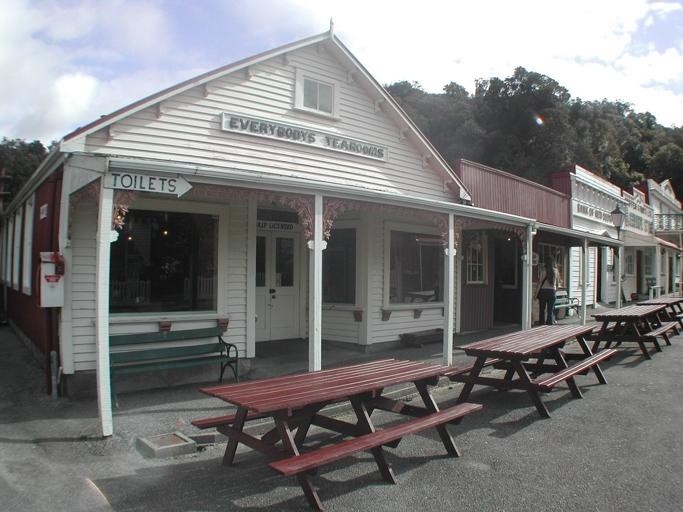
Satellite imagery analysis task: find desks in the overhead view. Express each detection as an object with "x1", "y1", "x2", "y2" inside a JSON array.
[
  {"x1": 635, "y1": 298, "x2": 682, "y2": 328},
  {"x1": 454, "y1": 324, "x2": 598, "y2": 419},
  {"x1": 590, "y1": 304, "x2": 666, "y2": 360},
  {"x1": 197, "y1": 358, "x2": 460, "y2": 512}
]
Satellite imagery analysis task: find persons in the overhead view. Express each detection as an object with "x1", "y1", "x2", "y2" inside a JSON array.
[{"x1": 535, "y1": 255, "x2": 560, "y2": 325}]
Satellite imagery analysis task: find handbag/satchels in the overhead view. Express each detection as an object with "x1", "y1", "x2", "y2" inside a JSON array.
[{"x1": 537, "y1": 288, "x2": 541, "y2": 300}]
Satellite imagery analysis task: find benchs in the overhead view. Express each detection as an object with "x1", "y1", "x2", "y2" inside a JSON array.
[
  {"x1": 440, "y1": 358, "x2": 508, "y2": 405},
  {"x1": 642, "y1": 321, "x2": 682, "y2": 360},
  {"x1": 190, "y1": 389, "x2": 384, "y2": 467},
  {"x1": 674, "y1": 313, "x2": 682, "y2": 328},
  {"x1": 591, "y1": 323, "x2": 624, "y2": 355},
  {"x1": 266, "y1": 401, "x2": 483, "y2": 512},
  {"x1": 550, "y1": 290, "x2": 582, "y2": 324},
  {"x1": 529, "y1": 348, "x2": 623, "y2": 419},
  {"x1": 108, "y1": 327, "x2": 239, "y2": 409}
]
